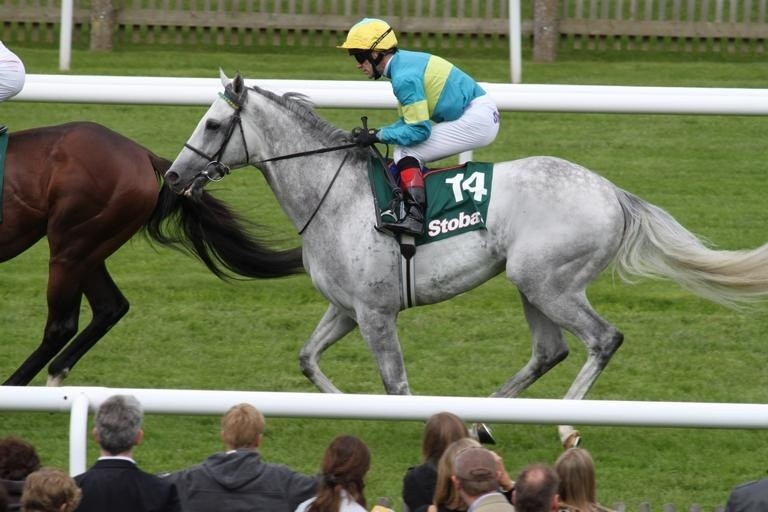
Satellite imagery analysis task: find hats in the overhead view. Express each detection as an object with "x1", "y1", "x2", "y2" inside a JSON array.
[{"x1": 454, "y1": 446, "x2": 498, "y2": 482}]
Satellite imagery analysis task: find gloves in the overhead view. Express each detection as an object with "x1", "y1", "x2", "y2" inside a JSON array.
[{"x1": 356, "y1": 128, "x2": 380, "y2": 148}]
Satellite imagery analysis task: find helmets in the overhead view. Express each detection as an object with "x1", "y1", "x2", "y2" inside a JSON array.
[{"x1": 335, "y1": 18, "x2": 398, "y2": 51}]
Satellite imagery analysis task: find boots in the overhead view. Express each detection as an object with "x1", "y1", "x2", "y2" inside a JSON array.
[{"x1": 382, "y1": 156, "x2": 427, "y2": 237}]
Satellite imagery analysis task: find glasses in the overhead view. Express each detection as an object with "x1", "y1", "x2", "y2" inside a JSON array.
[{"x1": 354, "y1": 52, "x2": 368, "y2": 64}]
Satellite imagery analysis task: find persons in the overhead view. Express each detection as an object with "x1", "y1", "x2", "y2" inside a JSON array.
[
  {"x1": 403, "y1": 411, "x2": 596, "y2": 512},
  {"x1": 333, "y1": 16, "x2": 501, "y2": 238},
  {"x1": 727, "y1": 480, "x2": 768, "y2": 511},
  {"x1": 22, "y1": 467, "x2": 82, "y2": 512},
  {"x1": 299, "y1": 434, "x2": 390, "y2": 512},
  {"x1": 0, "y1": 438, "x2": 43, "y2": 512},
  {"x1": 0, "y1": 37, "x2": 27, "y2": 103},
  {"x1": 161, "y1": 401, "x2": 318, "y2": 512},
  {"x1": 72, "y1": 395, "x2": 180, "y2": 511}
]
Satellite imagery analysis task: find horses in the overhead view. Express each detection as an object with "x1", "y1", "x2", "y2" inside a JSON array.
[
  {"x1": 0, "y1": 120, "x2": 307, "y2": 387},
  {"x1": 162, "y1": 66, "x2": 768, "y2": 449}
]
